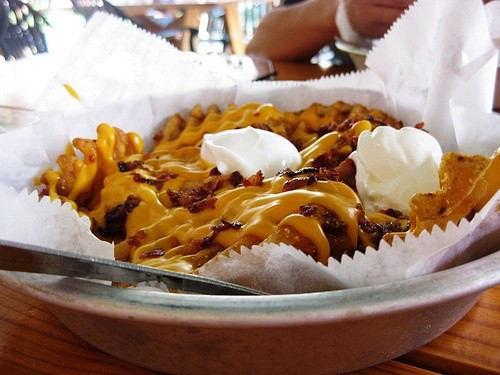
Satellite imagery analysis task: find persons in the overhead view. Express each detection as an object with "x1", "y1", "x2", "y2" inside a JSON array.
[{"x1": 246, "y1": 0, "x2": 500, "y2": 115}]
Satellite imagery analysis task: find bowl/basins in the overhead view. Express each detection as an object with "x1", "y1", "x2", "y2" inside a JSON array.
[
  {"x1": 334, "y1": 37, "x2": 378, "y2": 72},
  {"x1": 0, "y1": 83, "x2": 500, "y2": 375}
]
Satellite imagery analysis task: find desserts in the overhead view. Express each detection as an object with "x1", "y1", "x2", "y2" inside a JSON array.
[{"x1": 33, "y1": 96, "x2": 500, "y2": 293}]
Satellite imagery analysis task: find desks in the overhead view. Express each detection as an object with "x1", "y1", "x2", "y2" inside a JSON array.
[{"x1": 0, "y1": 60, "x2": 500, "y2": 375}]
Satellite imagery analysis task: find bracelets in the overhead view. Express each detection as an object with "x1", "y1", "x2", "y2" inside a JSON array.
[{"x1": 335, "y1": 0, "x2": 370, "y2": 47}]
